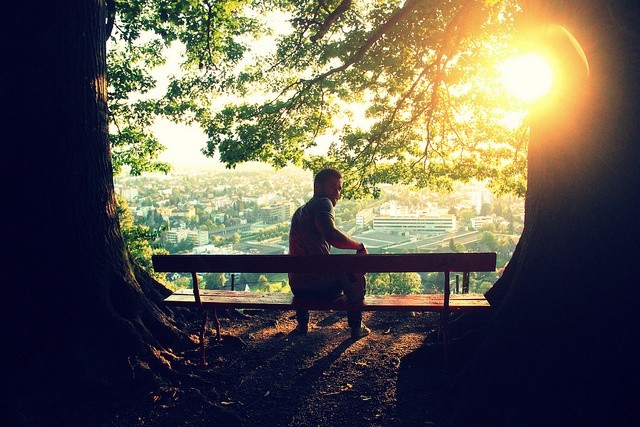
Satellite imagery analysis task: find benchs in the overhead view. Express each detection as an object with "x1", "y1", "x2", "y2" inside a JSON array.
[{"x1": 152, "y1": 252, "x2": 498, "y2": 365}]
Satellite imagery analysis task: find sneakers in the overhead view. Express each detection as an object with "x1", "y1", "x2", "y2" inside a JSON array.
[
  {"x1": 351, "y1": 324, "x2": 371, "y2": 338},
  {"x1": 296, "y1": 325, "x2": 308, "y2": 335}
]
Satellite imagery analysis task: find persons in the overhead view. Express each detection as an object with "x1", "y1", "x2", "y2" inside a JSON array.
[{"x1": 287, "y1": 168, "x2": 372, "y2": 339}]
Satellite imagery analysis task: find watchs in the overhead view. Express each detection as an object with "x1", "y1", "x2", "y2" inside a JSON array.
[{"x1": 356, "y1": 242, "x2": 366, "y2": 254}]
